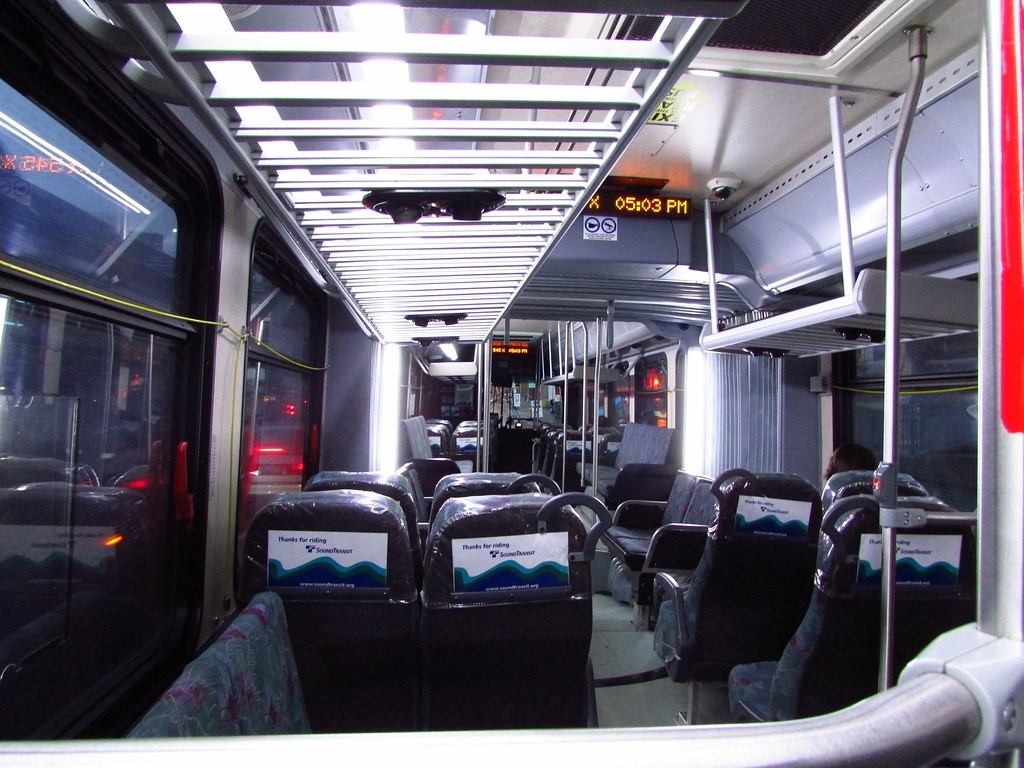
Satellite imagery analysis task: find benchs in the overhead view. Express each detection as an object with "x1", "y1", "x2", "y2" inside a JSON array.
[{"x1": 121, "y1": 590, "x2": 313, "y2": 738}]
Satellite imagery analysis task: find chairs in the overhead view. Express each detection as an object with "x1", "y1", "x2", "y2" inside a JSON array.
[
  {"x1": 241, "y1": 403, "x2": 979, "y2": 729},
  {"x1": 0, "y1": 454, "x2": 166, "y2": 663}
]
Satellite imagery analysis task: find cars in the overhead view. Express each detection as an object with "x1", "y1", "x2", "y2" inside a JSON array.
[
  {"x1": 107, "y1": 462, "x2": 195, "y2": 531},
  {"x1": 0, "y1": 480, "x2": 158, "y2": 604},
  {"x1": 0, "y1": 455, "x2": 100, "y2": 491},
  {"x1": 240, "y1": 424, "x2": 304, "y2": 496}
]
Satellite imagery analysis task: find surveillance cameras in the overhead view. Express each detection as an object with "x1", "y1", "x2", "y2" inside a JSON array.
[{"x1": 704, "y1": 177, "x2": 742, "y2": 199}]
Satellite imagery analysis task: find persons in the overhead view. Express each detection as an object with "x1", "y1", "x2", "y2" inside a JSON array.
[{"x1": 826, "y1": 443, "x2": 877, "y2": 480}]
